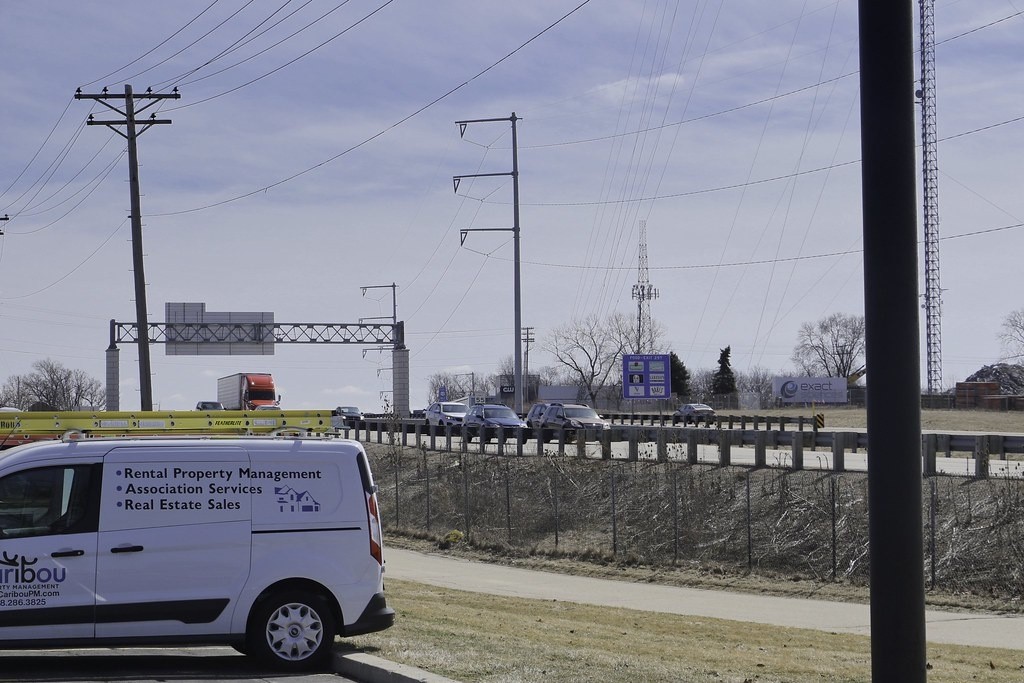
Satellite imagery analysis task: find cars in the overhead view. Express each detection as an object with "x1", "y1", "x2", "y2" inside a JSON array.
[
  {"x1": 335, "y1": 406, "x2": 365, "y2": 420},
  {"x1": 525, "y1": 402, "x2": 552, "y2": 430},
  {"x1": 253, "y1": 405, "x2": 283, "y2": 411},
  {"x1": 196, "y1": 400, "x2": 227, "y2": 411},
  {"x1": 673, "y1": 404, "x2": 715, "y2": 424}
]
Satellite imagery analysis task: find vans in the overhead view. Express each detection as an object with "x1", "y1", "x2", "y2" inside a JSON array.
[{"x1": 0, "y1": 428, "x2": 396, "y2": 672}]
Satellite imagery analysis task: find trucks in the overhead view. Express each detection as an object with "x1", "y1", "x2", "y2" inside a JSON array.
[{"x1": 217, "y1": 371, "x2": 281, "y2": 411}]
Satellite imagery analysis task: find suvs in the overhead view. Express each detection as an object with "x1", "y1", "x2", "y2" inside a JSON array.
[
  {"x1": 538, "y1": 403, "x2": 611, "y2": 443},
  {"x1": 422, "y1": 401, "x2": 472, "y2": 426},
  {"x1": 461, "y1": 402, "x2": 530, "y2": 443}
]
